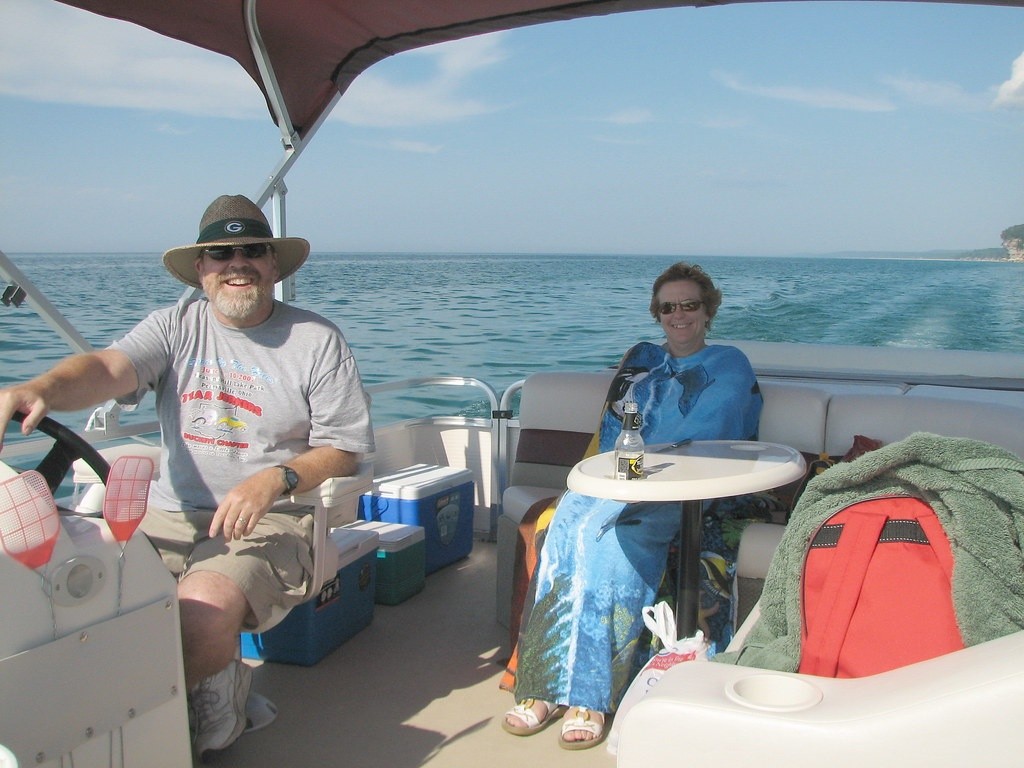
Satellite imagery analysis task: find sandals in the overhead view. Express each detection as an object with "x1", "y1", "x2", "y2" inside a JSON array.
[
  {"x1": 502, "y1": 698, "x2": 568, "y2": 736},
  {"x1": 559, "y1": 705, "x2": 606, "y2": 751}
]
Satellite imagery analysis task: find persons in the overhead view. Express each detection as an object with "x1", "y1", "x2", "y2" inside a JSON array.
[
  {"x1": 503, "y1": 260, "x2": 764, "y2": 751},
  {"x1": 0, "y1": 194, "x2": 375, "y2": 761}
]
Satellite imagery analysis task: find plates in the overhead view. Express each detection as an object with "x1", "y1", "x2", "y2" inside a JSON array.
[{"x1": 730, "y1": 444, "x2": 767, "y2": 452}]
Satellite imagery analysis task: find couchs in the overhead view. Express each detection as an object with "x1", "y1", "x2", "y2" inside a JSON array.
[{"x1": 495, "y1": 371, "x2": 1024, "y2": 652}]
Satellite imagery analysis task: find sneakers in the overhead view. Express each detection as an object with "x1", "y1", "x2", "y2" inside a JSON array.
[{"x1": 190, "y1": 659, "x2": 252, "y2": 764}]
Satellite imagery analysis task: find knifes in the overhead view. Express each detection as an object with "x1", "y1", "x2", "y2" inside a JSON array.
[{"x1": 655, "y1": 438, "x2": 692, "y2": 453}]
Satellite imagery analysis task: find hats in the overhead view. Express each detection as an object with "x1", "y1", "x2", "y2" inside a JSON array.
[{"x1": 162, "y1": 194, "x2": 310, "y2": 290}]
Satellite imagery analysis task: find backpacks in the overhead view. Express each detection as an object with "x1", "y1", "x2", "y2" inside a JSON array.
[{"x1": 796, "y1": 496, "x2": 963, "y2": 679}]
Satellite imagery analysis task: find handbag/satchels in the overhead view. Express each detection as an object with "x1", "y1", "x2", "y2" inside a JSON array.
[{"x1": 606, "y1": 601, "x2": 707, "y2": 756}]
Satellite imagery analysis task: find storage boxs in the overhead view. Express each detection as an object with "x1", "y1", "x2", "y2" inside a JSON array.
[
  {"x1": 240, "y1": 527, "x2": 380, "y2": 665},
  {"x1": 357, "y1": 463, "x2": 475, "y2": 576},
  {"x1": 339, "y1": 520, "x2": 426, "y2": 605}
]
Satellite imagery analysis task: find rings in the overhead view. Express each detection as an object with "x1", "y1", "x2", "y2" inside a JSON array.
[{"x1": 238, "y1": 518, "x2": 248, "y2": 524}]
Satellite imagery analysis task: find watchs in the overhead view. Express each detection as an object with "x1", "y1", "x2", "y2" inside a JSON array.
[{"x1": 274, "y1": 465, "x2": 298, "y2": 496}]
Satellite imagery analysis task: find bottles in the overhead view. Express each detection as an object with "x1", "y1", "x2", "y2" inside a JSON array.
[{"x1": 613, "y1": 402, "x2": 645, "y2": 482}]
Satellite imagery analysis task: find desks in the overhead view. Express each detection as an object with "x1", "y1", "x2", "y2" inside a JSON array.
[{"x1": 568, "y1": 440, "x2": 809, "y2": 641}]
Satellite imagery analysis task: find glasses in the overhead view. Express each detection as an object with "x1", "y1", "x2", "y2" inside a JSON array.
[
  {"x1": 657, "y1": 298, "x2": 705, "y2": 314},
  {"x1": 199, "y1": 243, "x2": 275, "y2": 260}
]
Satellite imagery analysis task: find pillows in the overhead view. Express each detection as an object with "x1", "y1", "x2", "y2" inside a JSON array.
[{"x1": 707, "y1": 451, "x2": 844, "y2": 525}]
